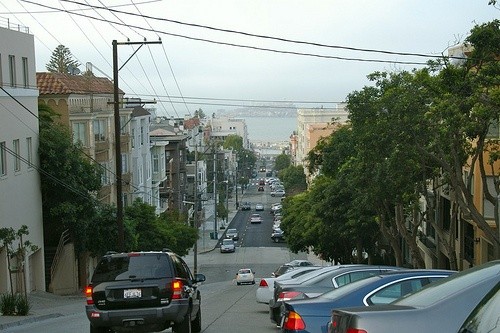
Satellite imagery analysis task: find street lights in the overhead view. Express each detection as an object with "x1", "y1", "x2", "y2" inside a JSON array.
[{"x1": 213, "y1": 180, "x2": 229, "y2": 239}]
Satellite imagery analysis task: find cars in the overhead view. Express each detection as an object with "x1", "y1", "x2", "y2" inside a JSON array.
[
  {"x1": 241, "y1": 201, "x2": 251, "y2": 211},
  {"x1": 219, "y1": 238, "x2": 236, "y2": 252},
  {"x1": 236, "y1": 268, "x2": 256, "y2": 285},
  {"x1": 260, "y1": 180, "x2": 265, "y2": 186},
  {"x1": 250, "y1": 213, "x2": 262, "y2": 223},
  {"x1": 259, "y1": 165, "x2": 266, "y2": 172},
  {"x1": 258, "y1": 187, "x2": 264, "y2": 191},
  {"x1": 256, "y1": 202, "x2": 265, "y2": 211},
  {"x1": 270, "y1": 202, "x2": 286, "y2": 243},
  {"x1": 225, "y1": 228, "x2": 240, "y2": 240},
  {"x1": 255, "y1": 259, "x2": 500, "y2": 333}
]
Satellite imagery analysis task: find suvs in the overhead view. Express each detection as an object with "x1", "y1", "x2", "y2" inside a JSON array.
[
  {"x1": 266, "y1": 178, "x2": 285, "y2": 197},
  {"x1": 84, "y1": 248, "x2": 206, "y2": 333}
]
